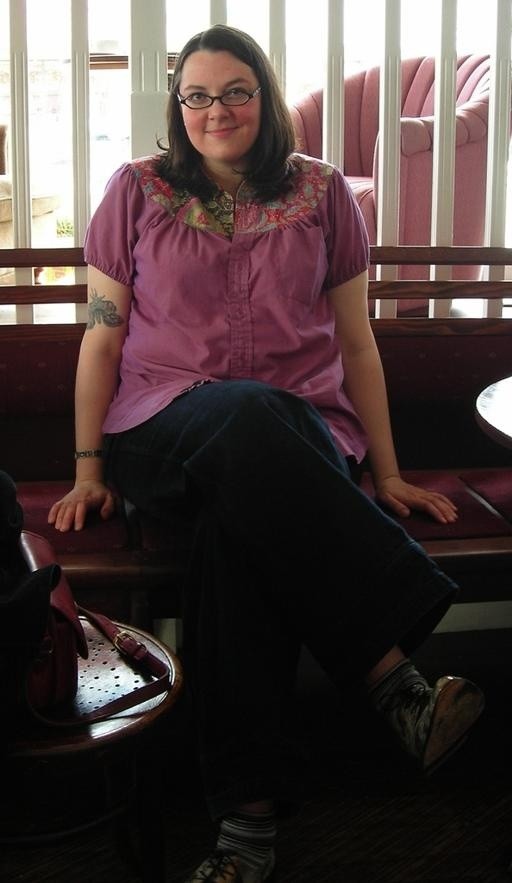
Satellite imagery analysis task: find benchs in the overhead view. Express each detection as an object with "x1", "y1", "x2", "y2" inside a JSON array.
[{"x1": 0, "y1": 244, "x2": 512, "y2": 622}]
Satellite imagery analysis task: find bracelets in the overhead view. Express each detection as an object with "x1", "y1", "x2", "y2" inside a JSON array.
[{"x1": 73, "y1": 449, "x2": 106, "y2": 460}]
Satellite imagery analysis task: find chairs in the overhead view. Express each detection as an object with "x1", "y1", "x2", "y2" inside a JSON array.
[{"x1": 287, "y1": 46, "x2": 498, "y2": 317}]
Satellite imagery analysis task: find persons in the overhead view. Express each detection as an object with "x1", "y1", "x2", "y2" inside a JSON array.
[{"x1": 47, "y1": 20, "x2": 494, "y2": 881}]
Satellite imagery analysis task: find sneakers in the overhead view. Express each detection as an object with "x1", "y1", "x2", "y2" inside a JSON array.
[
  {"x1": 184, "y1": 842, "x2": 282, "y2": 882},
  {"x1": 371, "y1": 670, "x2": 481, "y2": 781}
]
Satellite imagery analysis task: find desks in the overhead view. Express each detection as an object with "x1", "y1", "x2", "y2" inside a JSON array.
[{"x1": 0, "y1": 614, "x2": 183, "y2": 880}]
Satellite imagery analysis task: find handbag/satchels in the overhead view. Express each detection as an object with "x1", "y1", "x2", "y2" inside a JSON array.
[{"x1": 3, "y1": 520, "x2": 171, "y2": 740}]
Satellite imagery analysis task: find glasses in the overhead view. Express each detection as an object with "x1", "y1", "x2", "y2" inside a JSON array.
[{"x1": 173, "y1": 84, "x2": 264, "y2": 111}]
